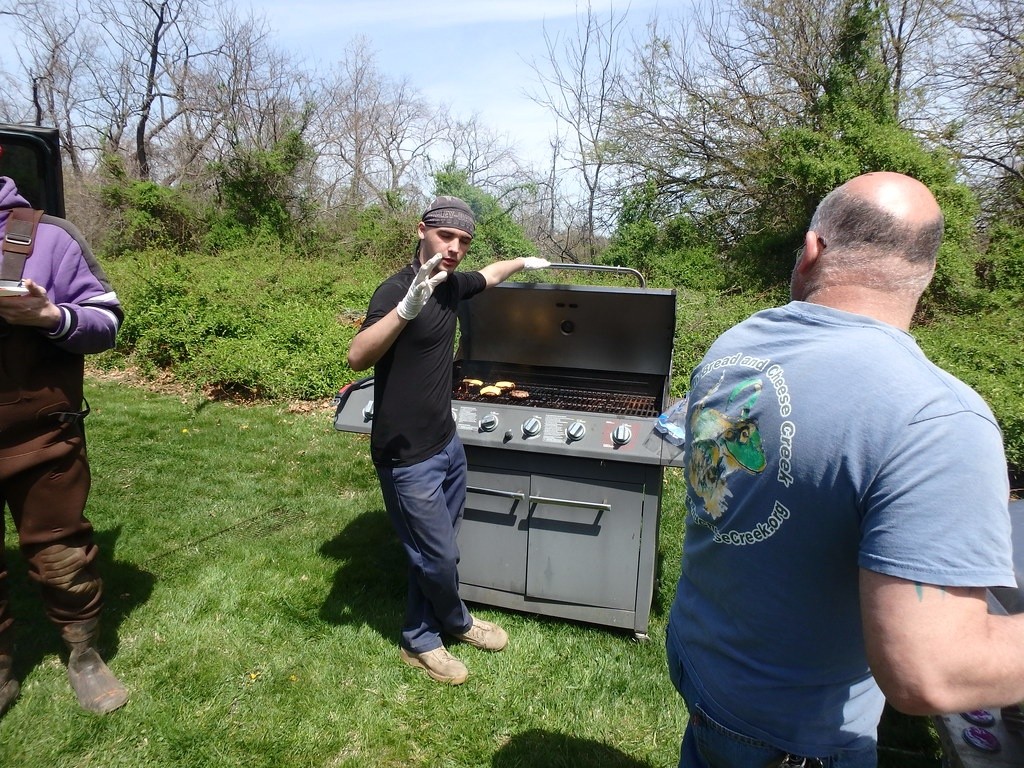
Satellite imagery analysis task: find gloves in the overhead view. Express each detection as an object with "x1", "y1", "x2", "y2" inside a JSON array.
[
  {"x1": 396, "y1": 254, "x2": 447, "y2": 320},
  {"x1": 519, "y1": 256, "x2": 551, "y2": 271}
]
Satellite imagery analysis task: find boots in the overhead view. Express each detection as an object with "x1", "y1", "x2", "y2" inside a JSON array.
[
  {"x1": 59, "y1": 623, "x2": 128, "y2": 715},
  {"x1": 0, "y1": 641, "x2": 20, "y2": 717}
]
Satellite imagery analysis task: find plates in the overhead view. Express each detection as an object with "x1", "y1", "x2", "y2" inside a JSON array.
[{"x1": 0, "y1": 281, "x2": 46, "y2": 296}]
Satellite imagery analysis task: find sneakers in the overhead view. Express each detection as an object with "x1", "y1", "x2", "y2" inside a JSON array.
[
  {"x1": 445, "y1": 613, "x2": 508, "y2": 651},
  {"x1": 399, "y1": 644, "x2": 468, "y2": 686}
]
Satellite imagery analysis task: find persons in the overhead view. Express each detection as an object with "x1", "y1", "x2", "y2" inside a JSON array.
[
  {"x1": 0, "y1": 175, "x2": 126, "y2": 713},
  {"x1": 346, "y1": 196, "x2": 550, "y2": 686},
  {"x1": 667, "y1": 172, "x2": 1024, "y2": 768}
]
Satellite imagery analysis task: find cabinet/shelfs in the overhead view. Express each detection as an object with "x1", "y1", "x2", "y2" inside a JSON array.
[{"x1": 457, "y1": 446, "x2": 661, "y2": 642}]
